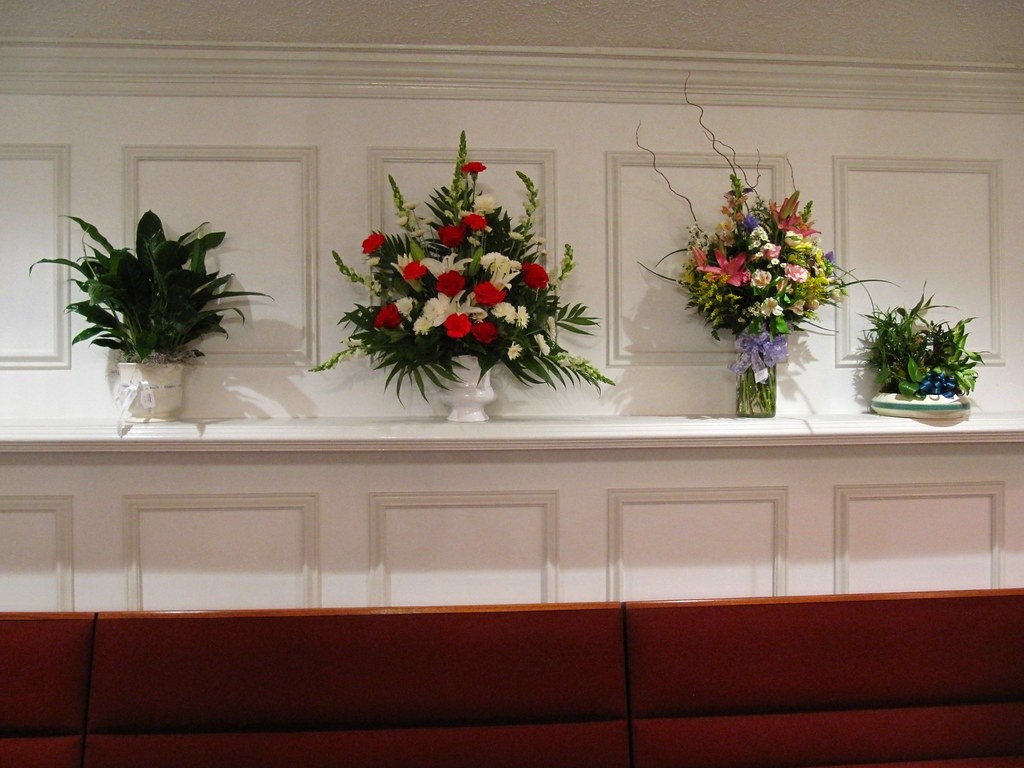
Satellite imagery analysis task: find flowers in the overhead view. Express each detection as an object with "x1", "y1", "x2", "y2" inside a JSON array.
[
  {"x1": 638, "y1": 177, "x2": 857, "y2": 336},
  {"x1": 310, "y1": 129, "x2": 616, "y2": 396}
]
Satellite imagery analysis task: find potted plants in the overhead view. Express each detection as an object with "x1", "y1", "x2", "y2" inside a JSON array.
[
  {"x1": 24, "y1": 210, "x2": 275, "y2": 423},
  {"x1": 863, "y1": 286, "x2": 988, "y2": 418}
]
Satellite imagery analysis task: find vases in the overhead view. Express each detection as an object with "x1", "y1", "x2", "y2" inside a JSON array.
[
  {"x1": 737, "y1": 335, "x2": 778, "y2": 418},
  {"x1": 434, "y1": 356, "x2": 495, "y2": 422}
]
